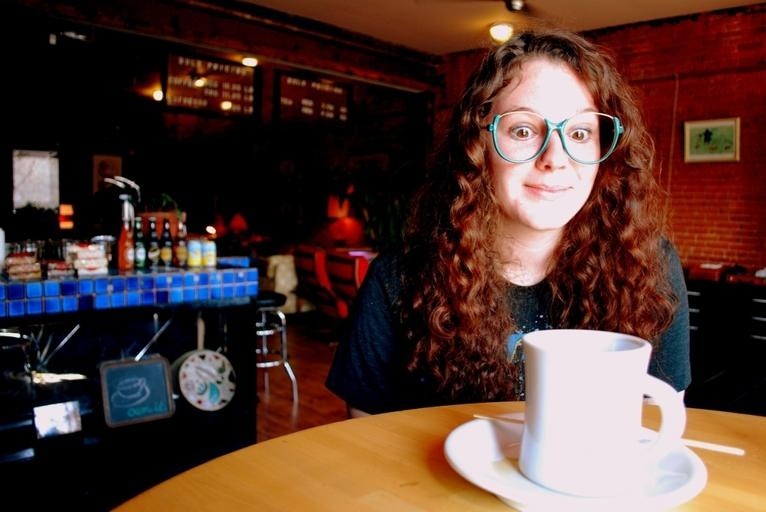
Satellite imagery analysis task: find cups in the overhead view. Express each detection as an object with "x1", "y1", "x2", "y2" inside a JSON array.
[{"x1": 516, "y1": 326, "x2": 688, "y2": 504}]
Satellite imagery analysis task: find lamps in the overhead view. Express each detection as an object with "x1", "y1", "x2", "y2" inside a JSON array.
[{"x1": 490, "y1": 23, "x2": 512, "y2": 43}]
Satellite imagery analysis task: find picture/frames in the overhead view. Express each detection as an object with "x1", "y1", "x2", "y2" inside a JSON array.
[{"x1": 684, "y1": 118, "x2": 740, "y2": 163}]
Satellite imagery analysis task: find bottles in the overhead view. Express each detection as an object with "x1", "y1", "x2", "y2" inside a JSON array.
[{"x1": 113, "y1": 193, "x2": 189, "y2": 271}]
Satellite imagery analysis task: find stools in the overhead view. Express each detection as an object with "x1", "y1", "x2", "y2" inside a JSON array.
[{"x1": 256, "y1": 290, "x2": 298, "y2": 401}]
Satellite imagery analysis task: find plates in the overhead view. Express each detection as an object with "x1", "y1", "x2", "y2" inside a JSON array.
[
  {"x1": 178, "y1": 349, "x2": 239, "y2": 412},
  {"x1": 440, "y1": 410, "x2": 710, "y2": 512}
]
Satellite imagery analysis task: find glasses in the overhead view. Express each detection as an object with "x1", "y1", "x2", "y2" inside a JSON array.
[{"x1": 478, "y1": 109, "x2": 624, "y2": 167}]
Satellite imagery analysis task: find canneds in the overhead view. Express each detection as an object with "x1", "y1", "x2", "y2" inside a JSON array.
[
  {"x1": 188, "y1": 240, "x2": 200, "y2": 267},
  {"x1": 202, "y1": 241, "x2": 216, "y2": 267}
]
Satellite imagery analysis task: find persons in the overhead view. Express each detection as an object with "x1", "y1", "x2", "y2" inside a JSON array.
[{"x1": 325, "y1": 27, "x2": 694, "y2": 418}]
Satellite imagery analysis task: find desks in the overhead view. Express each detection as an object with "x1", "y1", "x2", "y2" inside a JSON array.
[{"x1": 111, "y1": 401, "x2": 766, "y2": 511}]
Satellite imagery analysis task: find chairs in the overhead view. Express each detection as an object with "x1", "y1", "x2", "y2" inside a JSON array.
[{"x1": 295, "y1": 245, "x2": 370, "y2": 317}]
